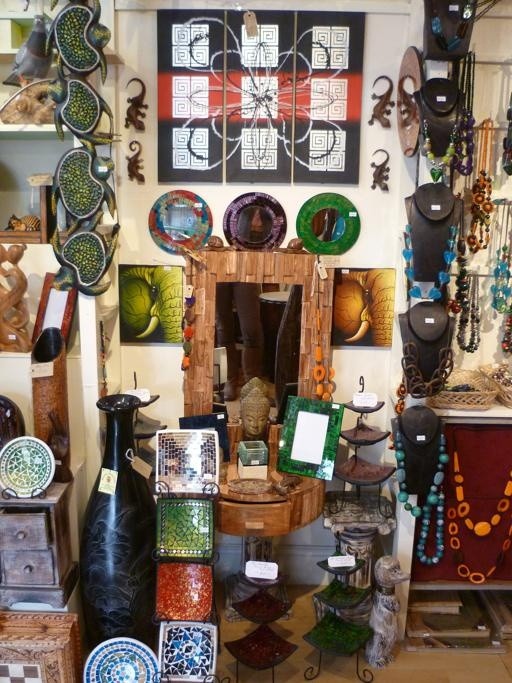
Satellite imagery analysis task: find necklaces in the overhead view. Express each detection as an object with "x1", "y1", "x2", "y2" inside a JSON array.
[
  {"x1": 467, "y1": 118, "x2": 493, "y2": 254},
  {"x1": 450, "y1": 447, "x2": 512, "y2": 539},
  {"x1": 502, "y1": 311, "x2": 511, "y2": 352},
  {"x1": 415, "y1": 488, "x2": 449, "y2": 566},
  {"x1": 389, "y1": 424, "x2": 450, "y2": 521},
  {"x1": 491, "y1": 199, "x2": 510, "y2": 313},
  {"x1": 418, "y1": 90, "x2": 460, "y2": 183},
  {"x1": 402, "y1": 314, "x2": 453, "y2": 397},
  {"x1": 447, "y1": 200, "x2": 470, "y2": 313},
  {"x1": 431, "y1": 2, "x2": 473, "y2": 51},
  {"x1": 402, "y1": 192, "x2": 458, "y2": 299},
  {"x1": 443, "y1": 487, "x2": 511, "y2": 586},
  {"x1": 456, "y1": 272, "x2": 480, "y2": 353},
  {"x1": 502, "y1": 96, "x2": 511, "y2": 175},
  {"x1": 454, "y1": 51, "x2": 475, "y2": 176}
]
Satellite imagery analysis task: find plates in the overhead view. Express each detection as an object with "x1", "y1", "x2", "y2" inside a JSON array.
[
  {"x1": 82, "y1": 637, "x2": 159, "y2": 683},
  {"x1": 0, "y1": 437, "x2": 54, "y2": 499}
]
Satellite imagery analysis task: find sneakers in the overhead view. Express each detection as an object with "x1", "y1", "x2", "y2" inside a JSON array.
[
  {"x1": 224, "y1": 382, "x2": 235, "y2": 399},
  {"x1": 266, "y1": 396, "x2": 276, "y2": 407}
]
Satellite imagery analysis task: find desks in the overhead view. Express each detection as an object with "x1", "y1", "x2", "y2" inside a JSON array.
[{"x1": 215, "y1": 460, "x2": 327, "y2": 624}]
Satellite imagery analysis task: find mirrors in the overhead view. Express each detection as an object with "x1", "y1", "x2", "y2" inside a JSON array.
[{"x1": 181, "y1": 251, "x2": 335, "y2": 465}]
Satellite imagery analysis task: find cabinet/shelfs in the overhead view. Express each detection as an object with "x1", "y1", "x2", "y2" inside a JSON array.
[
  {"x1": 1, "y1": 10, "x2": 120, "y2": 361},
  {"x1": 0, "y1": 478, "x2": 83, "y2": 611}
]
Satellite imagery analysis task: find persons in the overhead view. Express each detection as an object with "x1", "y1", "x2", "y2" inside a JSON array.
[
  {"x1": 237, "y1": 374, "x2": 271, "y2": 404},
  {"x1": 239, "y1": 388, "x2": 272, "y2": 439},
  {"x1": 212, "y1": 281, "x2": 277, "y2": 410}
]
